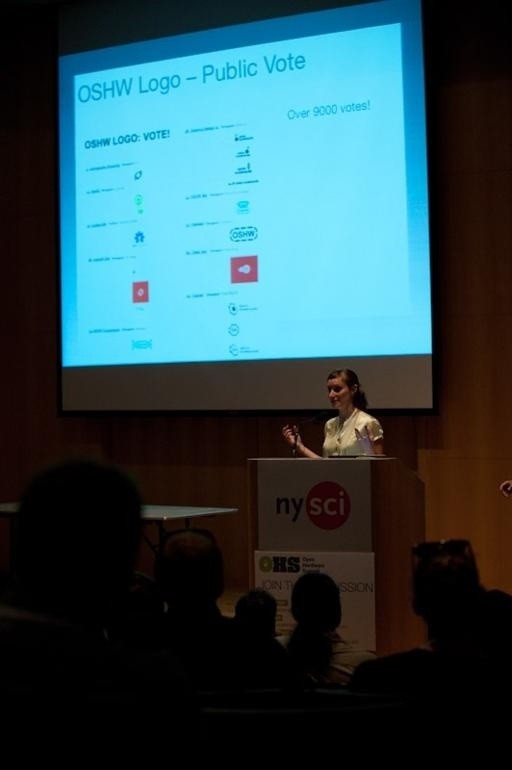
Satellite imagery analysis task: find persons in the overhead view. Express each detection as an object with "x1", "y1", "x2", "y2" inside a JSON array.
[
  {"x1": 282, "y1": 367, "x2": 387, "y2": 458},
  {"x1": 1, "y1": 456, "x2": 512, "y2": 769}
]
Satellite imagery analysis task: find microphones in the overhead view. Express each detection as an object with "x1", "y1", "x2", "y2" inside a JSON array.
[{"x1": 296, "y1": 412, "x2": 331, "y2": 425}]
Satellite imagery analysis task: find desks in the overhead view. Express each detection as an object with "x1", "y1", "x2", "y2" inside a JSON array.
[{"x1": 0, "y1": 499, "x2": 242, "y2": 587}]
